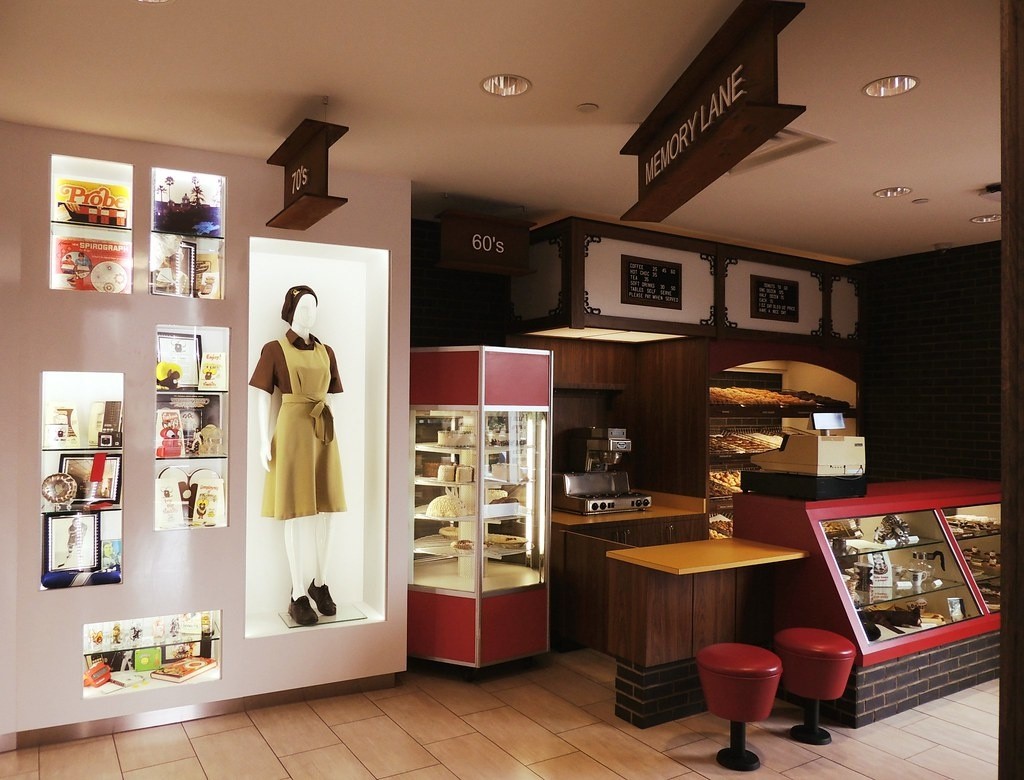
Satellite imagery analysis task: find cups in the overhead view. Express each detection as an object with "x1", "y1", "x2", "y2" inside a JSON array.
[
  {"x1": 908, "y1": 569, "x2": 927, "y2": 587},
  {"x1": 46, "y1": 425, "x2": 68, "y2": 449},
  {"x1": 833, "y1": 538, "x2": 846, "y2": 554}
]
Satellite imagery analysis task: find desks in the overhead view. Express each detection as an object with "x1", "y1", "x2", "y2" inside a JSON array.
[{"x1": 606, "y1": 537, "x2": 811, "y2": 730}]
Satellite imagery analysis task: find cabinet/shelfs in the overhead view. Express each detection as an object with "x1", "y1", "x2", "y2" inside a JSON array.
[
  {"x1": 409, "y1": 346, "x2": 552, "y2": 668},
  {"x1": 708, "y1": 385, "x2": 857, "y2": 539},
  {"x1": 584, "y1": 514, "x2": 709, "y2": 547}
]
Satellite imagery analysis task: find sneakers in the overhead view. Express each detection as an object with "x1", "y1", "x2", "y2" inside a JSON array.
[
  {"x1": 288, "y1": 594, "x2": 319, "y2": 625},
  {"x1": 308, "y1": 579, "x2": 336, "y2": 616}
]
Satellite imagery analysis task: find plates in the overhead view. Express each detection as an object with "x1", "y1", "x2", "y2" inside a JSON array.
[
  {"x1": 90, "y1": 261, "x2": 127, "y2": 293},
  {"x1": 42, "y1": 473, "x2": 77, "y2": 502}
]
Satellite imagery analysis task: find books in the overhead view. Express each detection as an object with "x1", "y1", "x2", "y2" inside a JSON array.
[
  {"x1": 150, "y1": 656, "x2": 218, "y2": 683},
  {"x1": 110, "y1": 672, "x2": 146, "y2": 688}
]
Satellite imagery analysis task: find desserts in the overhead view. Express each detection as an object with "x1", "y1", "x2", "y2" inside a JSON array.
[
  {"x1": 439, "y1": 526, "x2": 528, "y2": 554},
  {"x1": 945, "y1": 516, "x2": 1003, "y2": 577}
]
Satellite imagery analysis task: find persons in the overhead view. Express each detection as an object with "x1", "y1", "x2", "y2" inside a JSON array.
[
  {"x1": 157, "y1": 248, "x2": 189, "y2": 294},
  {"x1": 103, "y1": 542, "x2": 116, "y2": 566},
  {"x1": 57, "y1": 518, "x2": 87, "y2": 567},
  {"x1": 248, "y1": 285, "x2": 348, "y2": 626},
  {"x1": 191, "y1": 428, "x2": 202, "y2": 450},
  {"x1": 102, "y1": 487, "x2": 109, "y2": 498},
  {"x1": 77, "y1": 475, "x2": 89, "y2": 499}
]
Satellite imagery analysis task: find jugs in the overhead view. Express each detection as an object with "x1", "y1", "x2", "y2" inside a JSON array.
[{"x1": 912, "y1": 550, "x2": 945, "y2": 582}]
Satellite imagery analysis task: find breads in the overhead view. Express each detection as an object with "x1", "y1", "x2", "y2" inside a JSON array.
[
  {"x1": 709, "y1": 386, "x2": 851, "y2": 408},
  {"x1": 709, "y1": 435, "x2": 776, "y2": 539}
]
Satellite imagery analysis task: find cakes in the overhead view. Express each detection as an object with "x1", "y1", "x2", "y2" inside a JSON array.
[
  {"x1": 486, "y1": 462, "x2": 520, "y2": 516},
  {"x1": 425, "y1": 430, "x2": 474, "y2": 518}
]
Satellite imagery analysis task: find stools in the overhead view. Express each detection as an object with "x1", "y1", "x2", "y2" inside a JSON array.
[
  {"x1": 772, "y1": 627, "x2": 857, "y2": 745},
  {"x1": 696, "y1": 642, "x2": 783, "y2": 773}
]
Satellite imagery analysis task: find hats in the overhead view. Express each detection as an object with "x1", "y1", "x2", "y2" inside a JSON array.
[{"x1": 281, "y1": 285, "x2": 318, "y2": 326}]
